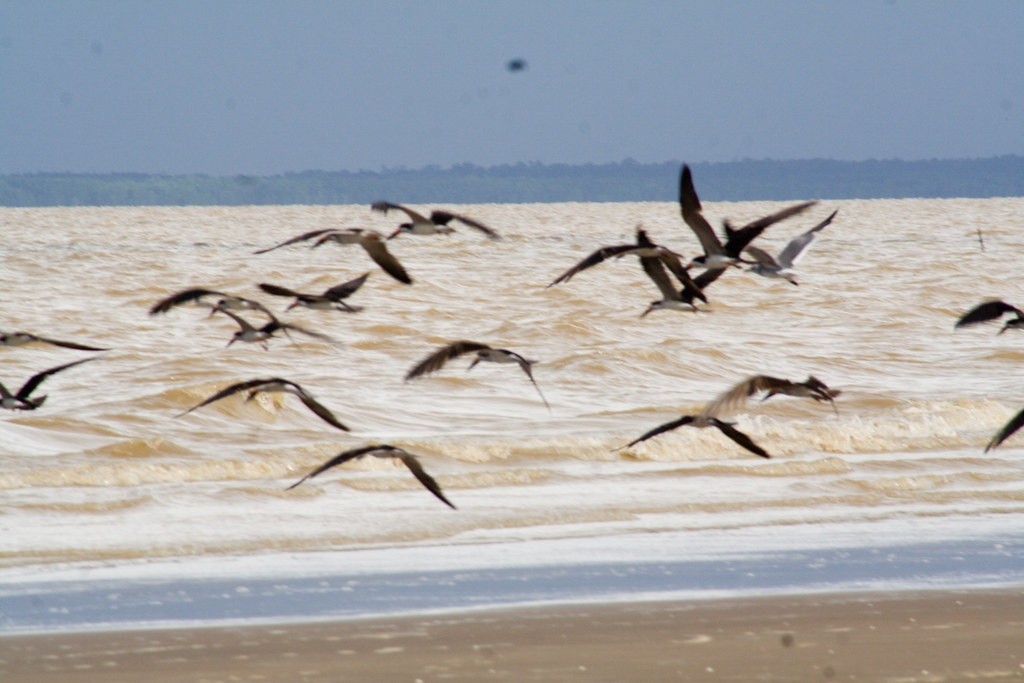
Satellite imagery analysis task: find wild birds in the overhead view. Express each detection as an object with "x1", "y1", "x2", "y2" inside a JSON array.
[
  {"x1": 253, "y1": 227, "x2": 413, "y2": 286},
  {"x1": 610, "y1": 374, "x2": 842, "y2": 460},
  {"x1": 402, "y1": 338, "x2": 540, "y2": 385},
  {"x1": 955, "y1": 299, "x2": 1024, "y2": 335},
  {"x1": 175, "y1": 377, "x2": 350, "y2": 432},
  {"x1": 0, "y1": 330, "x2": 111, "y2": 410},
  {"x1": 285, "y1": 444, "x2": 459, "y2": 511},
  {"x1": 372, "y1": 200, "x2": 502, "y2": 240},
  {"x1": 544, "y1": 162, "x2": 838, "y2": 319},
  {"x1": 149, "y1": 286, "x2": 335, "y2": 352},
  {"x1": 257, "y1": 271, "x2": 371, "y2": 313}
]
